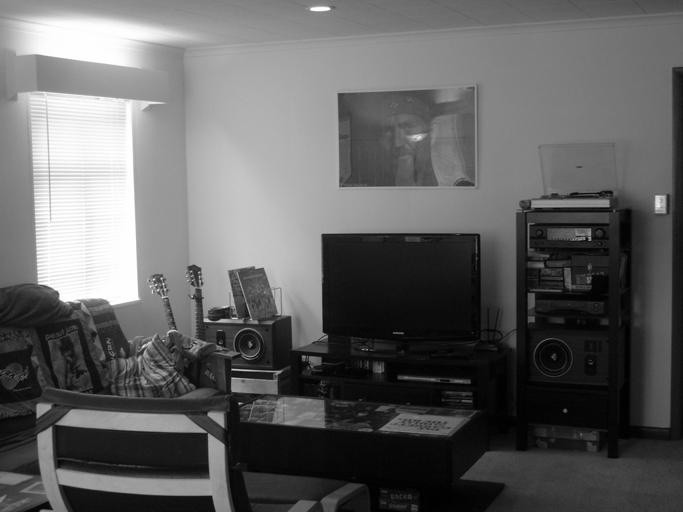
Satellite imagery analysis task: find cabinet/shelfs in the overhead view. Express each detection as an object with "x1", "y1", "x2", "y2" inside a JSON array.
[
  {"x1": 289, "y1": 335, "x2": 513, "y2": 435},
  {"x1": 517, "y1": 207, "x2": 631, "y2": 450},
  {"x1": 230, "y1": 360, "x2": 309, "y2": 396}
]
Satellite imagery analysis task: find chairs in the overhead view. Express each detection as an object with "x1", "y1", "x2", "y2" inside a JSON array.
[
  {"x1": 32, "y1": 385, "x2": 370, "y2": 512},
  {"x1": 1, "y1": 297, "x2": 241, "y2": 475}
]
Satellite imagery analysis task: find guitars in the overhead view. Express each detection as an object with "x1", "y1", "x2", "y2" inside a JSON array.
[
  {"x1": 147, "y1": 273, "x2": 176, "y2": 331},
  {"x1": 185, "y1": 265, "x2": 205, "y2": 341}
]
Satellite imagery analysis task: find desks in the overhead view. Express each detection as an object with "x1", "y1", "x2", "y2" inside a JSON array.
[{"x1": 238, "y1": 394, "x2": 505, "y2": 494}]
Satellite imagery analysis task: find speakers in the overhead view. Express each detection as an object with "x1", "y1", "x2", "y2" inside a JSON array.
[
  {"x1": 204, "y1": 315, "x2": 291, "y2": 370},
  {"x1": 528, "y1": 322, "x2": 609, "y2": 386}
]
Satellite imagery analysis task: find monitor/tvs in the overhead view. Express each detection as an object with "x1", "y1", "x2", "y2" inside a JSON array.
[{"x1": 321, "y1": 233, "x2": 481, "y2": 354}]
